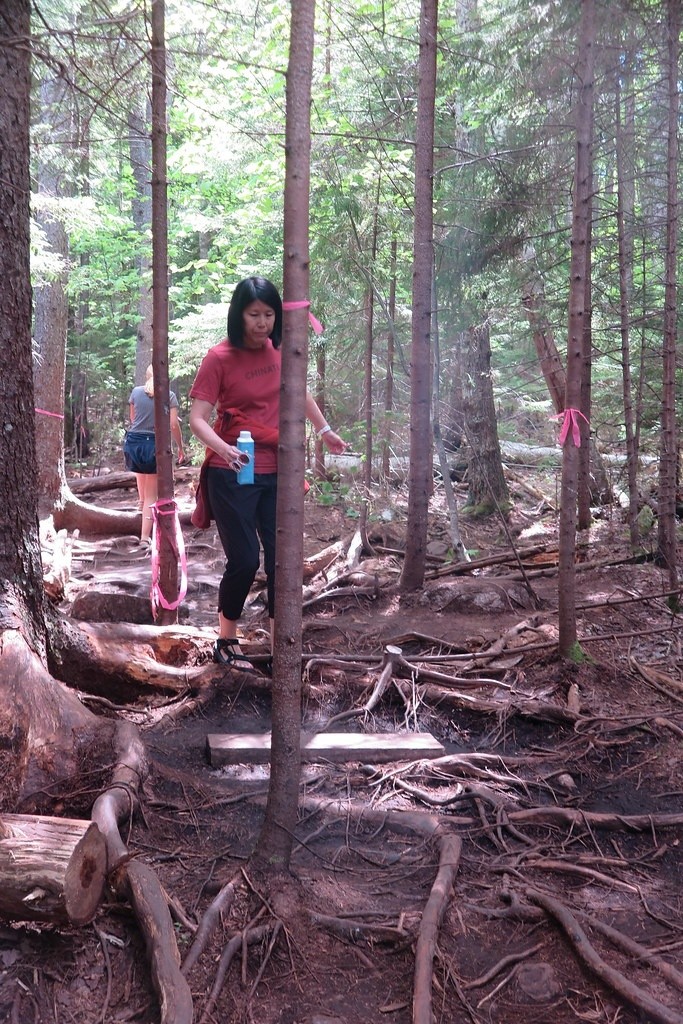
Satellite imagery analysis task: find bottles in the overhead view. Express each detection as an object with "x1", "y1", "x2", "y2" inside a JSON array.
[{"x1": 237, "y1": 430, "x2": 255, "y2": 485}]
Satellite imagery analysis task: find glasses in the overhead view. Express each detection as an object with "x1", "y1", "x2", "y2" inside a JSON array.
[{"x1": 229, "y1": 450, "x2": 256, "y2": 472}]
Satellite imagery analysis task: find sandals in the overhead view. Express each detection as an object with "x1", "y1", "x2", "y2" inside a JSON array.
[
  {"x1": 268, "y1": 656, "x2": 273, "y2": 669},
  {"x1": 138, "y1": 501, "x2": 144, "y2": 515},
  {"x1": 212, "y1": 638, "x2": 255, "y2": 672},
  {"x1": 140, "y1": 537, "x2": 152, "y2": 549}
]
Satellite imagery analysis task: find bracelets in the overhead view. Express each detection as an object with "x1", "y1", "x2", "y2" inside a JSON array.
[{"x1": 317, "y1": 425, "x2": 331, "y2": 435}]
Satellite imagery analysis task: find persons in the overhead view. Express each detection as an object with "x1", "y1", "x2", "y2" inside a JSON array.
[
  {"x1": 123, "y1": 364, "x2": 185, "y2": 546},
  {"x1": 188, "y1": 276, "x2": 349, "y2": 675}
]
